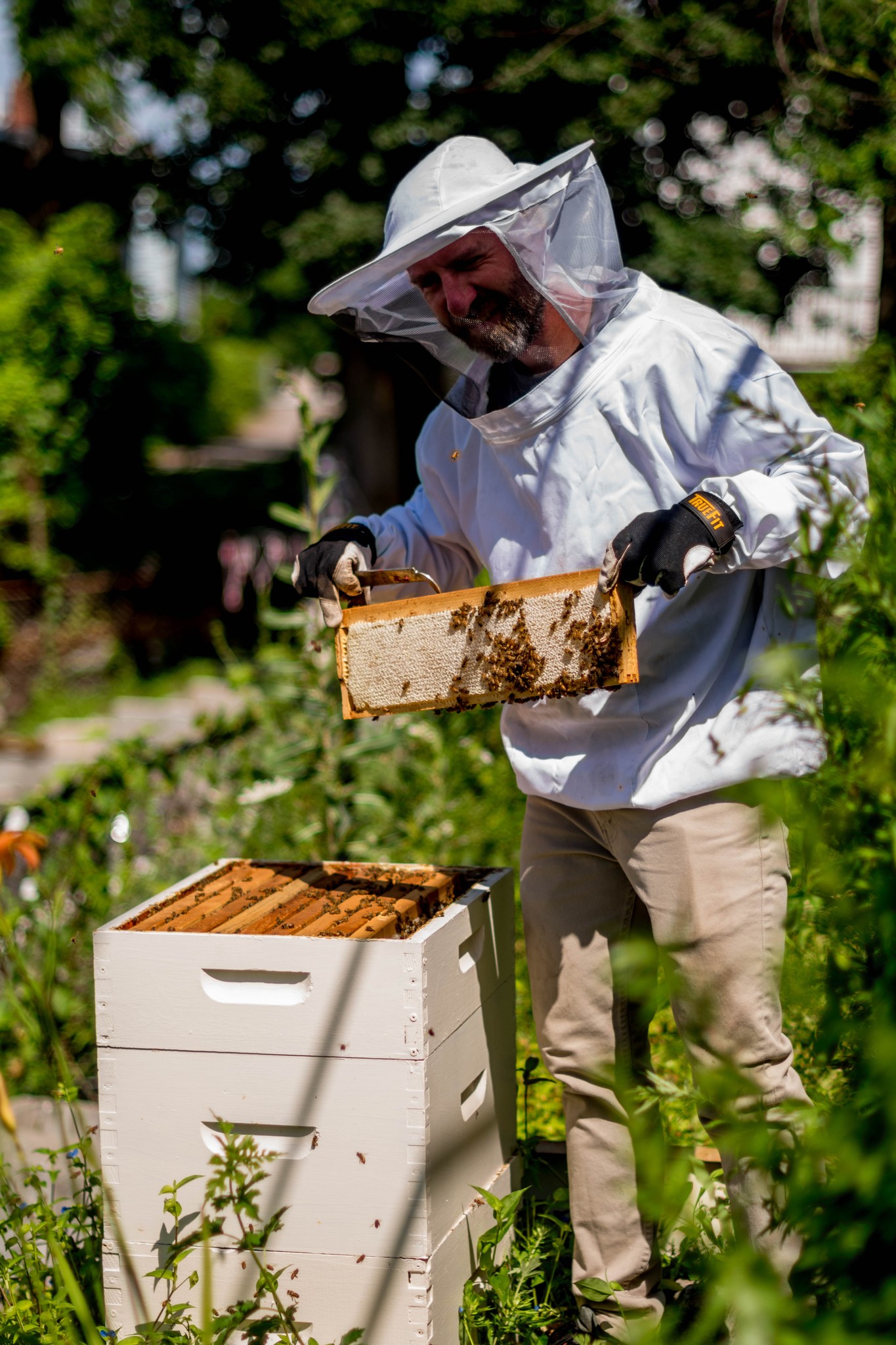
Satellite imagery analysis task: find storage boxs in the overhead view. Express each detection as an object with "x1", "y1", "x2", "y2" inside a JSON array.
[{"x1": 93, "y1": 847, "x2": 532, "y2": 1345}]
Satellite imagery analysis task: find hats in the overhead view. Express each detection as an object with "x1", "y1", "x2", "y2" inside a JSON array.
[{"x1": 308, "y1": 134, "x2": 595, "y2": 314}]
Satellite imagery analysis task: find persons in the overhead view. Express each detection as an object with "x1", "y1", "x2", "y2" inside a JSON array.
[{"x1": 310, "y1": 135, "x2": 868, "y2": 1345}]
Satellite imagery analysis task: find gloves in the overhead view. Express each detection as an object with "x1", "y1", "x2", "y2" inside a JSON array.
[
  {"x1": 291, "y1": 523, "x2": 376, "y2": 629},
  {"x1": 596, "y1": 491, "x2": 741, "y2": 600}
]
